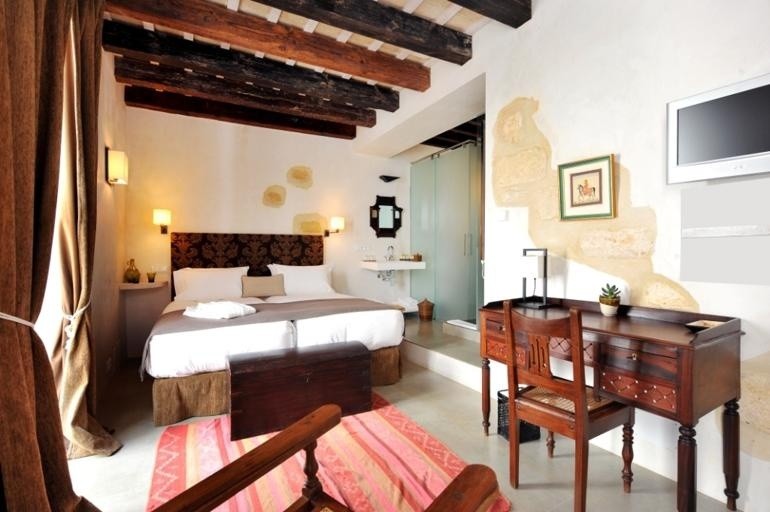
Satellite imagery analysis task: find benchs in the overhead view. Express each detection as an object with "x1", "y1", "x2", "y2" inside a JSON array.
[{"x1": 225, "y1": 340, "x2": 373, "y2": 441}]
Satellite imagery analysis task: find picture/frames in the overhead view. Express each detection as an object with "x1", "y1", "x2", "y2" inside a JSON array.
[{"x1": 557, "y1": 154, "x2": 618, "y2": 220}]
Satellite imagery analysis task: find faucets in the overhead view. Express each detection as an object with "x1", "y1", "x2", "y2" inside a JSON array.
[{"x1": 388, "y1": 244, "x2": 394, "y2": 260}]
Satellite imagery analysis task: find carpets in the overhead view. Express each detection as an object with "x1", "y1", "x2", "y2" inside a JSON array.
[
  {"x1": 447, "y1": 319, "x2": 477, "y2": 331},
  {"x1": 146, "y1": 387, "x2": 512, "y2": 512}
]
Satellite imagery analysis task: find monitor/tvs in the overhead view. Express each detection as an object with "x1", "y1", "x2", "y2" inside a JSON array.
[{"x1": 667, "y1": 74, "x2": 770, "y2": 185}]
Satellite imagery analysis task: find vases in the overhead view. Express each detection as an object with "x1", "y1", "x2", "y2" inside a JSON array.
[{"x1": 125, "y1": 259, "x2": 140, "y2": 283}]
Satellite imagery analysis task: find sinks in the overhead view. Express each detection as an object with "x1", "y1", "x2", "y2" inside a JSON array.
[{"x1": 360, "y1": 259, "x2": 426, "y2": 271}]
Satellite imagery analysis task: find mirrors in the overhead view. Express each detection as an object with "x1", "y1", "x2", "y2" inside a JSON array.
[{"x1": 370, "y1": 195, "x2": 403, "y2": 238}]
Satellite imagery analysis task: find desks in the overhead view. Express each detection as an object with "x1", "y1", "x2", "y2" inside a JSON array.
[{"x1": 479, "y1": 295, "x2": 742, "y2": 511}]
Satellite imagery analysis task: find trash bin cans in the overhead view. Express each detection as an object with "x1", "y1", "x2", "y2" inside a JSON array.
[
  {"x1": 417, "y1": 298, "x2": 434, "y2": 323},
  {"x1": 497, "y1": 387, "x2": 541, "y2": 443}
]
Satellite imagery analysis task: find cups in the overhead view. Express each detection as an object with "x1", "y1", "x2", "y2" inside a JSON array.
[{"x1": 147, "y1": 272, "x2": 157, "y2": 282}]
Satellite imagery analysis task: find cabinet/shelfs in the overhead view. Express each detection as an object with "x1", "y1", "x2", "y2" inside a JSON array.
[{"x1": 360, "y1": 259, "x2": 426, "y2": 314}]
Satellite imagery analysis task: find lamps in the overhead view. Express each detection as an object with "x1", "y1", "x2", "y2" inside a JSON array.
[
  {"x1": 324, "y1": 215, "x2": 345, "y2": 237},
  {"x1": 153, "y1": 208, "x2": 171, "y2": 234},
  {"x1": 518, "y1": 247, "x2": 552, "y2": 310},
  {"x1": 104, "y1": 145, "x2": 129, "y2": 186}
]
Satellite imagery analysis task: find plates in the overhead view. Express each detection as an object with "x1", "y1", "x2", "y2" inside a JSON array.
[{"x1": 687, "y1": 319, "x2": 726, "y2": 331}]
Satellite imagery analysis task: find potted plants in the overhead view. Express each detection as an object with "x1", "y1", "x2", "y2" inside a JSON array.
[{"x1": 599, "y1": 283, "x2": 623, "y2": 316}]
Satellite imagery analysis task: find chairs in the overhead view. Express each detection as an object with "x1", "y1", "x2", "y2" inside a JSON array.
[
  {"x1": 503, "y1": 297, "x2": 634, "y2": 512},
  {"x1": 151, "y1": 403, "x2": 498, "y2": 512}
]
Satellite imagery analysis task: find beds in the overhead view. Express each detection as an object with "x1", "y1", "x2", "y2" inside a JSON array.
[{"x1": 141, "y1": 232, "x2": 404, "y2": 423}]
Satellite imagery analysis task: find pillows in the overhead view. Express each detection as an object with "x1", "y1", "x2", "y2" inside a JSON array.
[{"x1": 173, "y1": 264, "x2": 336, "y2": 298}]
